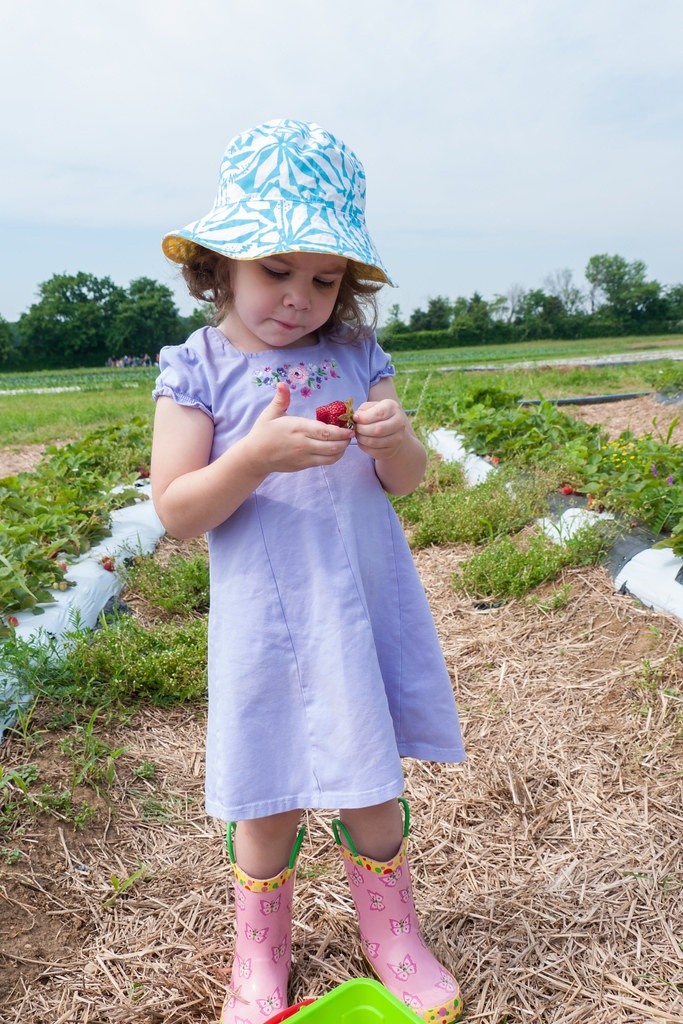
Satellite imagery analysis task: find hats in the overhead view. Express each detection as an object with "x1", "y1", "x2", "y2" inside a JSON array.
[{"x1": 161, "y1": 118, "x2": 399, "y2": 289}]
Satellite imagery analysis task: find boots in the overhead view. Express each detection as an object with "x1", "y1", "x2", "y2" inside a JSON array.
[
  {"x1": 219, "y1": 820, "x2": 306, "y2": 1024},
  {"x1": 332, "y1": 797, "x2": 464, "y2": 1024}
]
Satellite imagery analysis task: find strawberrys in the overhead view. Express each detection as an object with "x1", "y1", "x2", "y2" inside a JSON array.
[{"x1": 0, "y1": 401, "x2": 683, "y2": 628}]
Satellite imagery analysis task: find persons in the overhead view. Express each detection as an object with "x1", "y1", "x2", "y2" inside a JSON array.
[
  {"x1": 118, "y1": 353, "x2": 151, "y2": 366},
  {"x1": 152, "y1": 118, "x2": 464, "y2": 1024}
]
